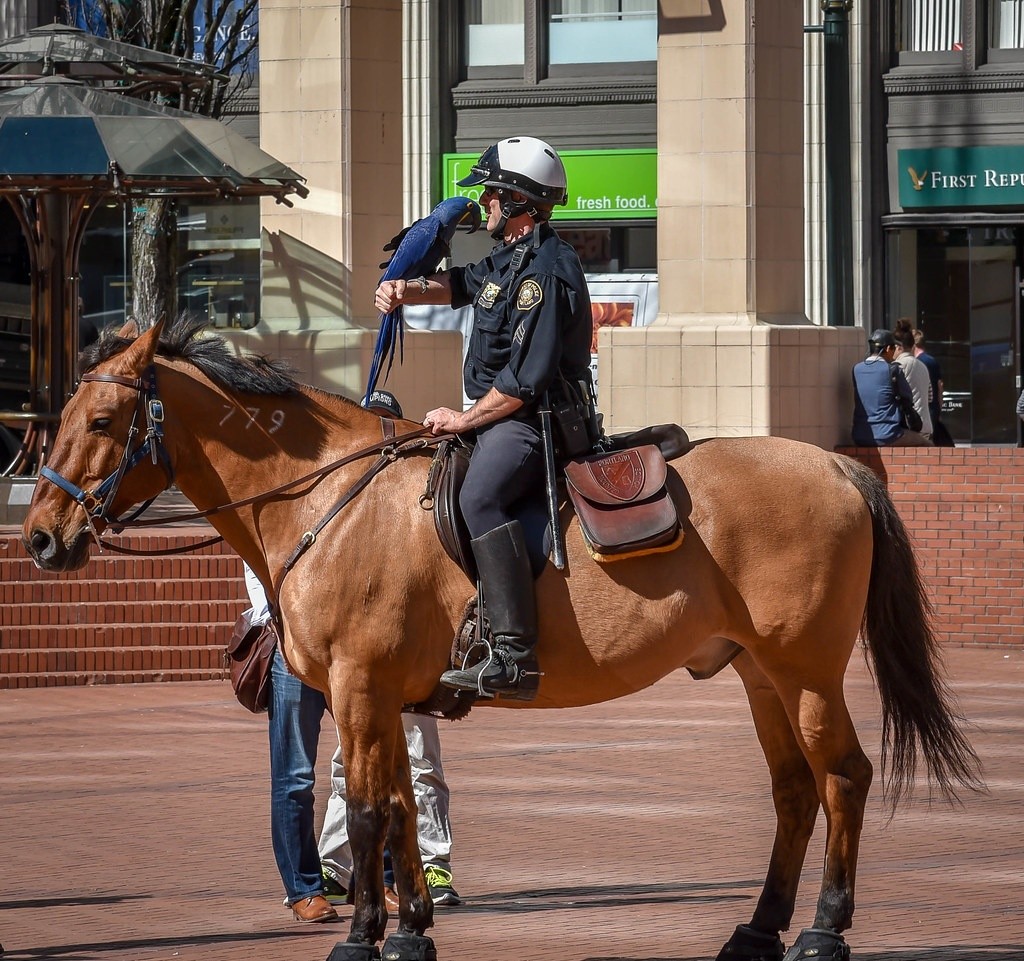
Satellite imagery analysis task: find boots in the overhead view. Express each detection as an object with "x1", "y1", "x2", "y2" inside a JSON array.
[{"x1": 439, "y1": 516, "x2": 542, "y2": 702}]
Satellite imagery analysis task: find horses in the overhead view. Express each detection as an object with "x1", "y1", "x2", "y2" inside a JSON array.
[{"x1": 23, "y1": 316, "x2": 990, "y2": 961}]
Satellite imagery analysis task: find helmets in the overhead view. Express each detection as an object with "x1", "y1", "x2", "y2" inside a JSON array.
[{"x1": 456, "y1": 136, "x2": 569, "y2": 206}]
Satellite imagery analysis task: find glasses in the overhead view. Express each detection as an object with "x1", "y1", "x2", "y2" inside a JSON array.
[{"x1": 484, "y1": 185, "x2": 496, "y2": 197}]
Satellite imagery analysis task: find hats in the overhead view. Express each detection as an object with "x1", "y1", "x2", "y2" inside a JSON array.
[{"x1": 868, "y1": 329, "x2": 904, "y2": 348}]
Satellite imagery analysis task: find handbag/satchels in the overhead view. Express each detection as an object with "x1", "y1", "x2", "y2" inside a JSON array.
[
  {"x1": 220, "y1": 613, "x2": 278, "y2": 714},
  {"x1": 888, "y1": 363, "x2": 924, "y2": 433},
  {"x1": 559, "y1": 447, "x2": 687, "y2": 562}
]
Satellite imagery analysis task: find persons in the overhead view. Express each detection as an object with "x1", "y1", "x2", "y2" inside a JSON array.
[
  {"x1": 890, "y1": 318, "x2": 932, "y2": 440},
  {"x1": 281, "y1": 391, "x2": 459, "y2": 905},
  {"x1": 852, "y1": 330, "x2": 932, "y2": 448},
  {"x1": 374, "y1": 135, "x2": 594, "y2": 698},
  {"x1": 243, "y1": 561, "x2": 401, "y2": 923},
  {"x1": 910, "y1": 330, "x2": 937, "y2": 412}
]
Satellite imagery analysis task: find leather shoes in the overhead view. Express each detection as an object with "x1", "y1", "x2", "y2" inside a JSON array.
[
  {"x1": 292, "y1": 895, "x2": 338, "y2": 922},
  {"x1": 384, "y1": 886, "x2": 402, "y2": 918}
]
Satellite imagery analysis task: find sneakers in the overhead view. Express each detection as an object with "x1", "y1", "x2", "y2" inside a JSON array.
[
  {"x1": 282, "y1": 867, "x2": 349, "y2": 905},
  {"x1": 424, "y1": 865, "x2": 461, "y2": 905}
]
